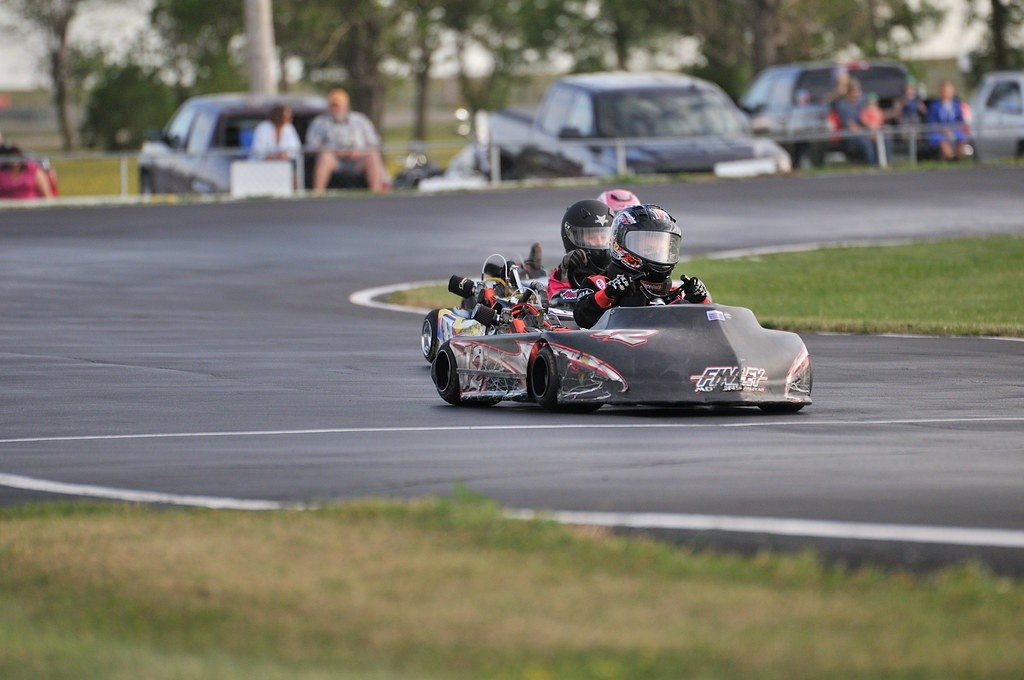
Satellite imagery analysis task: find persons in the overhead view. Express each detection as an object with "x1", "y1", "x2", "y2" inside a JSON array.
[
  {"x1": 572, "y1": 204, "x2": 711, "y2": 329},
  {"x1": 925, "y1": 80, "x2": 974, "y2": 162},
  {"x1": 249, "y1": 101, "x2": 301, "y2": 190},
  {"x1": 597, "y1": 190, "x2": 641, "y2": 214},
  {"x1": 834, "y1": 79, "x2": 903, "y2": 167},
  {"x1": 0, "y1": 147, "x2": 55, "y2": 201},
  {"x1": 546, "y1": 198, "x2": 616, "y2": 303},
  {"x1": 305, "y1": 89, "x2": 384, "y2": 193},
  {"x1": 893, "y1": 84, "x2": 928, "y2": 139},
  {"x1": 786, "y1": 90, "x2": 829, "y2": 169}
]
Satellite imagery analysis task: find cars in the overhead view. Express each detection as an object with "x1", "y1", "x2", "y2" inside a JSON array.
[
  {"x1": 732, "y1": 59, "x2": 929, "y2": 169},
  {"x1": 140, "y1": 90, "x2": 330, "y2": 197},
  {"x1": 967, "y1": 70, "x2": 1024, "y2": 160}
]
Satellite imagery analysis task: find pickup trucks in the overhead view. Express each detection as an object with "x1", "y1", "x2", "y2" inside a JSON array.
[{"x1": 473, "y1": 66, "x2": 795, "y2": 179}]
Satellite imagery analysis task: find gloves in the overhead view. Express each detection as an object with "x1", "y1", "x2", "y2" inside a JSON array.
[
  {"x1": 680, "y1": 277, "x2": 707, "y2": 303},
  {"x1": 606, "y1": 273, "x2": 635, "y2": 299},
  {"x1": 561, "y1": 248, "x2": 587, "y2": 271}
]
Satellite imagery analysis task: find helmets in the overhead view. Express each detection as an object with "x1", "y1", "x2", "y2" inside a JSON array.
[
  {"x1": 561, "y1": 199, "x2": 615, "y2": 267},
  {"x1": 609, "y1": 204, "x2": 682, "y2": 281},
  {"x1": 597, "y1": 190, "x2": 640, "y2": 212}
]
